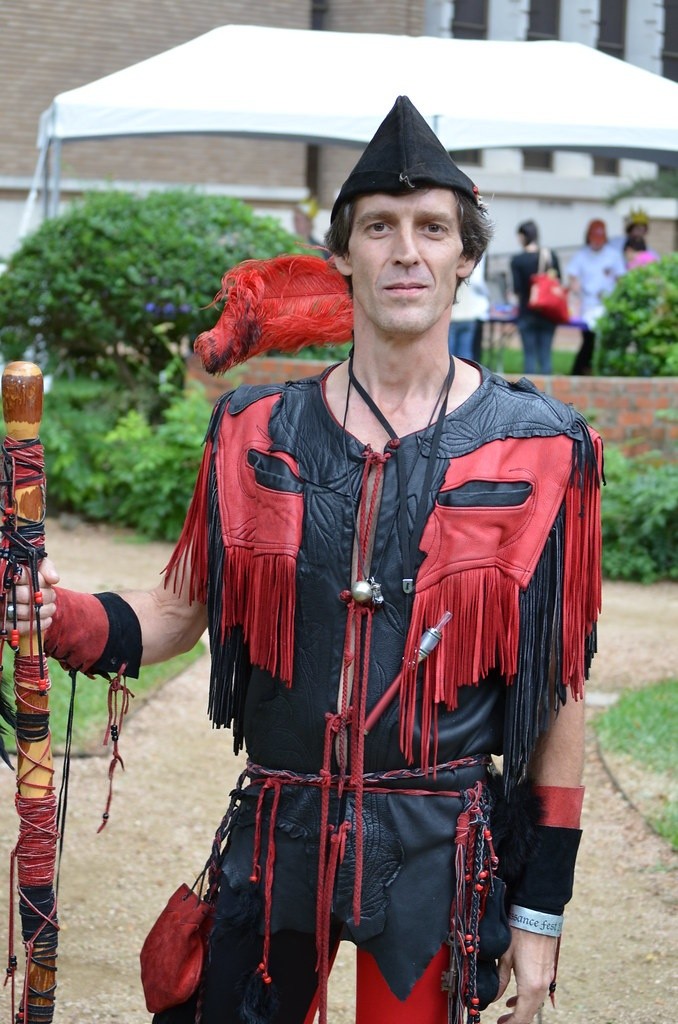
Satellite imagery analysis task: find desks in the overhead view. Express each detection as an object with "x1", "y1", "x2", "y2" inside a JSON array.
[{"x1": 485, "y1": 315, "x2": 586, "y2": 374}]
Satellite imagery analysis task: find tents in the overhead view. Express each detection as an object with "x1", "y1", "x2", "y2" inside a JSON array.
[{"x1": 16, "y1": 24, "x2": 678, "y2": 247}]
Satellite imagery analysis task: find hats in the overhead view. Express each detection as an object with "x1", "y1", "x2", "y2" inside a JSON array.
[{"x1": 330, "y1": 94, "x2": 481, "y2": 225}]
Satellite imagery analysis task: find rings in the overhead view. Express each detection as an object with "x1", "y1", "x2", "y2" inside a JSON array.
[
  {"x1": 6, "y1": 603, "x2": 17, "y2": 621},
  {"x1": 538, "y1": 1002, "x2": 545, "y2": 1009}
]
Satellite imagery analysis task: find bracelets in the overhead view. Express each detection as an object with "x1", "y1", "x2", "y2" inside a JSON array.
[{"x1": 508, "y1": 903, "x2": 564, "y2": 938}]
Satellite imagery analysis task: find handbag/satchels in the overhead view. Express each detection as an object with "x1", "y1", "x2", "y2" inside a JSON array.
[{"x1": 529, "y1": 247, "x2": 569, "y2": 323}]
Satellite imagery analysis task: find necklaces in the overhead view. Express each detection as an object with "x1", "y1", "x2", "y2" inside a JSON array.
[{"x1": 340, "y1": 347, "x2": 457, "y2": 610}]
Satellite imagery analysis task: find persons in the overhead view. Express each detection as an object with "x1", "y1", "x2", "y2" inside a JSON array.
[
  {"x1": 0, "y1": 95, "x2": 602, "y2": 1024},
  {"x1": 449, "y1": 211, "x2": 656, "y2": 376}
]
